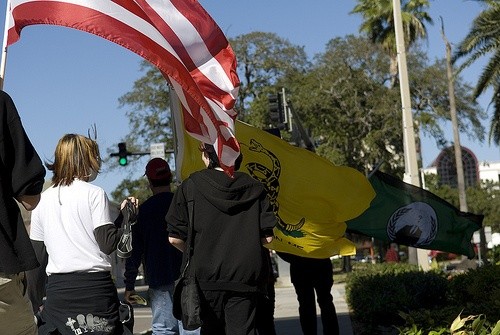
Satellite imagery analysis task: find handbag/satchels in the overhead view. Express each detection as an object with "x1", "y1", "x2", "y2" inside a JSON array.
[{"x1": 172, "y1": 275, "x2": 204, "y2": 331}]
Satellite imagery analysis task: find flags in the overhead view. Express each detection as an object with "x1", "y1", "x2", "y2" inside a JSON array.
[
  {"x1": 178, "y1": 100, "x2": 376, "y2": 257},
  {"x1": 5, "y1": 0, "x2": 240, "y2": 180},
  {"x1": 345, "y1": 169, "x2": 484, "y2": 260}
]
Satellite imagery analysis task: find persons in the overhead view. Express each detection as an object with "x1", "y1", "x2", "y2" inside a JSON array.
[
  {"x1": 275, "y1": 250, "x2": 340, "y2": 335},
  {"x1": 0, "y1": 90, "x2": 46, "y2": 335},
  {"x1": 26, "y1": 133, "x2": 139, "y2": 335},
  {"x1": 166, "y1": 143, "x2": 278, "y2": 335},
  {"x1": 124, "y1": 158, "x2": 201, "y2": 335}
]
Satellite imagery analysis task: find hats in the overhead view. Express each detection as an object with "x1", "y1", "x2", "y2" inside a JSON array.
[{"x1": 142, "y1": 157, "x2": 171, "y2": 179}]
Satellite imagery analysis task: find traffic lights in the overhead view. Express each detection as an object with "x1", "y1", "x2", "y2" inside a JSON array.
[{"x1": 117, "y1": 142, "x2": 128, "y2": 167}]
